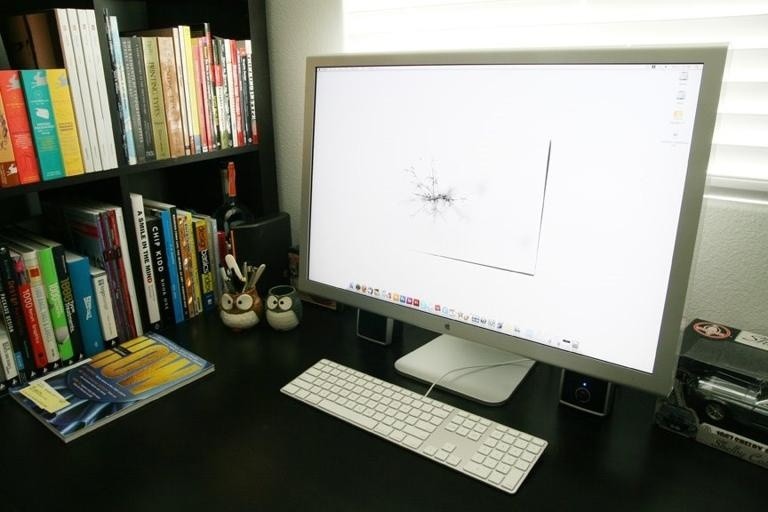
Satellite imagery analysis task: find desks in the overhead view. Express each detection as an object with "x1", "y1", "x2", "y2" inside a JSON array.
[{"x1": 1, "y1": 287, "x2": 768, "y2": 511}]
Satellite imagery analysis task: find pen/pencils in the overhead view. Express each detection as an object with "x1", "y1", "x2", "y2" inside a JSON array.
[{"x1": 219, "y1": 254, "x2": 266, "y2": 295}]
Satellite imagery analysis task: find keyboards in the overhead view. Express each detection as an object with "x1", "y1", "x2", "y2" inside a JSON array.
[{"x1": 279, "y1": 357, "x2": 549, "y2": 495}]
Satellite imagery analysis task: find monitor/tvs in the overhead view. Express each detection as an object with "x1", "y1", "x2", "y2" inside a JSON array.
[{"x1": 297, "y1": 43, "x2": 729, "y2": 407}]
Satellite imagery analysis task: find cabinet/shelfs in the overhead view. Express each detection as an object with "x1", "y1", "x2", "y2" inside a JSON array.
[{"x1": 1, "y1": 1, "x2": 277, "y2": 392}]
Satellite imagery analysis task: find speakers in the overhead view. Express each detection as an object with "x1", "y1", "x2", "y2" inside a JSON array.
[
  {"x1": 356, "y1": 307, "x2": 404, "y2": 346},
  {"x1": 558, "y1": 367, "x2": 616, "y2": 417}
]
